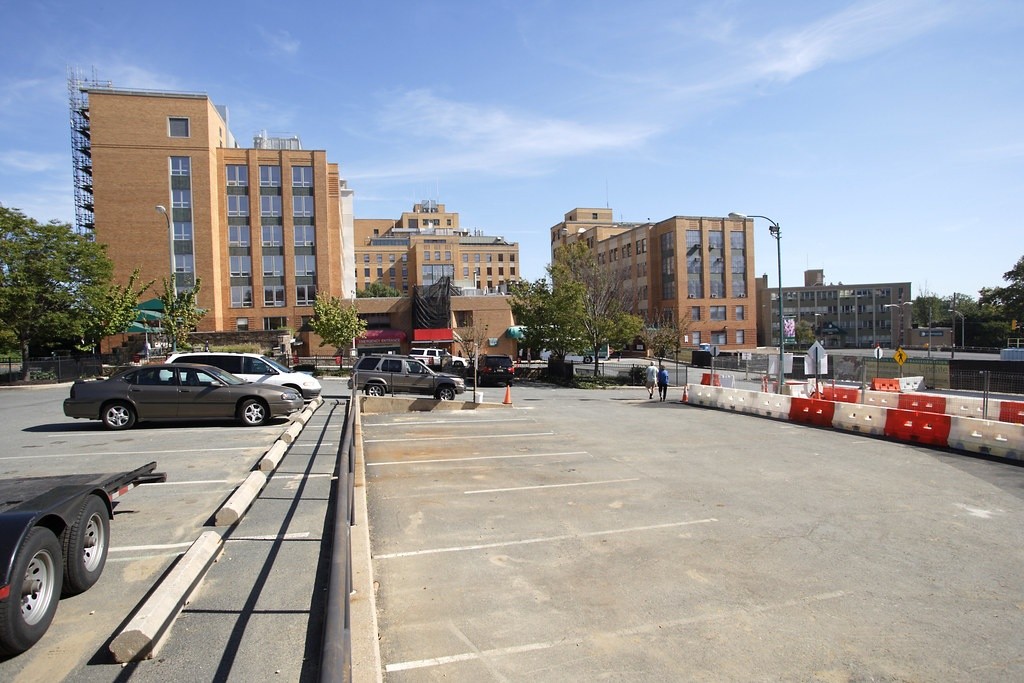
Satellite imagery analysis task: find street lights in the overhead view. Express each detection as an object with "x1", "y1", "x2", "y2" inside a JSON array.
[
  {"x1": 882, "y1": 300, "x2": 914, "y2": 349},
  {"x1": 947, "y1": 310, "x2": 965, "y2": 349},
  {"x1": 728, "y1": 212, "x2": 786, "y2": 397},
  {"x1": 814, "y1": 313, "x2": 822, "y2": 344}
]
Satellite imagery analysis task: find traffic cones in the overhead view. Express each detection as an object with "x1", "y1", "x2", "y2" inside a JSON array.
[
  {"x1": 679, "y1": 385, "x2": 688, "y2": 403},
  {"x1": 503, "y1": 385, "x2": 513, "y2": 404}
]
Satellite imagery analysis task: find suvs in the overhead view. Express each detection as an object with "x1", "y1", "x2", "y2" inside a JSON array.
[
  {"x1": 477, "y1": 352, "x2": 515, "y2": 388},
  {"x1": 408, "y1": 347, "x2": 471, "y2": 371},
  {"x1": 158, "y1": 351, "x2": 322, "y2": 404},
  {"x1": 347, "y1": 353, "x2": 467, "y2": 401}
]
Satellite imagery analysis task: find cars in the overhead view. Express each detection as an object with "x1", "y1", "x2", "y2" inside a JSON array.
[
  {"x1": 542, "y1": 347, "x2": 594, "y2": 364},
  {"x1": 63, "y1": 361, "x2": 305, "y2": 431}
]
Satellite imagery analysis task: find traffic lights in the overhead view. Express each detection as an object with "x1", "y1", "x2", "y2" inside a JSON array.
[{"x1": 1011, "y1": 319, "x2": 1017, "y2": 331}]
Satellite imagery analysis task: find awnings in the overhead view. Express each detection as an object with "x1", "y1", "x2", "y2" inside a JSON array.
[
  {"x1": 356, "y1": 329, "x2": 407, "y2": 343},
  {"x1": 506, "y1": 325, "x2": 528, "y2": 339}
]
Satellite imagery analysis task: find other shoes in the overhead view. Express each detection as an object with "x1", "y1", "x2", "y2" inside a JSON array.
[
  {"x1": 649, "y1": 393, "x2": 652, "y2": 399},
  {"x1": 660, "y1": 397, "x2": 662, "y2": 402},
  {"x1": 663, "y1": 398, "x2": 665, "y2": 401}
]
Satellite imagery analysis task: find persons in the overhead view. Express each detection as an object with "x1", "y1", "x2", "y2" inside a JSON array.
[
  {"x1": 657, "y1": 365, "x2": 669, "y2": 402},
  {"x1": 644, "y1": 361, "x2": 659, "y2": 399}
]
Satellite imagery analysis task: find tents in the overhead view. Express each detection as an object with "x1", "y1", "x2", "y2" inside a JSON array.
[{"x1": 116, "y1": 298, "x2": 171, "y2": 364}]
